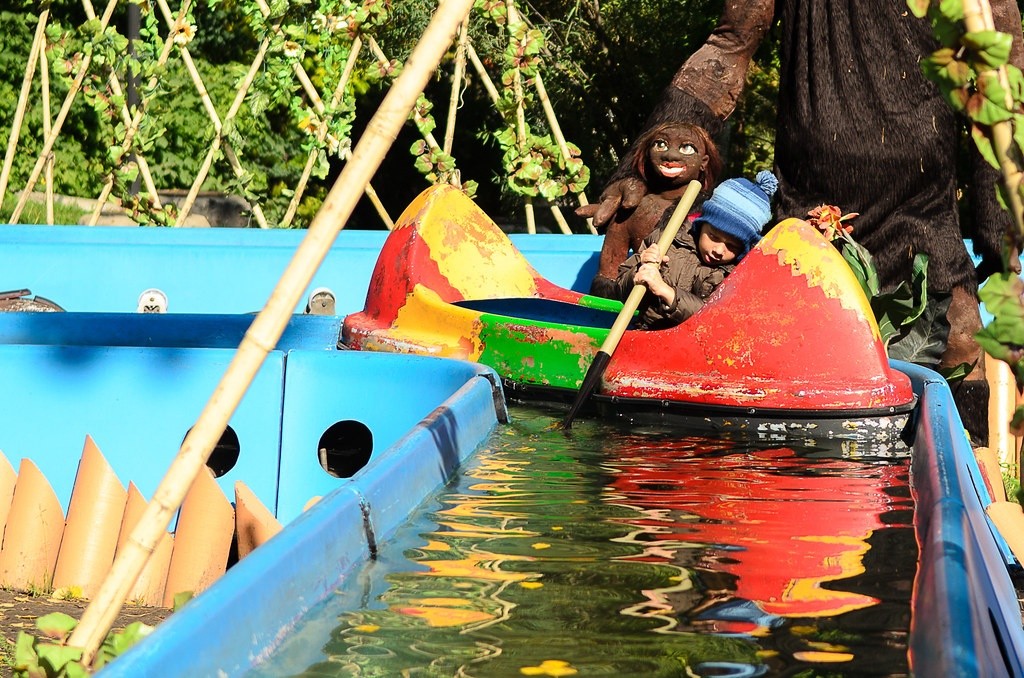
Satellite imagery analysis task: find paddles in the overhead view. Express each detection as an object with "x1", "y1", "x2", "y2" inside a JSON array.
[{"x1": 556, "y1": 177, "x2": 703, "y2": 432}]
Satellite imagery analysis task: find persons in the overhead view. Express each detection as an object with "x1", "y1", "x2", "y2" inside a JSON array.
[{"x1": 615, "y1": 170, "x2": 779, "y2": 332}]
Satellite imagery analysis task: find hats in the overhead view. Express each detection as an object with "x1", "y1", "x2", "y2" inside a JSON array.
[{"x1": 692, "y1": 170, "x2": 779, "y2": 260}]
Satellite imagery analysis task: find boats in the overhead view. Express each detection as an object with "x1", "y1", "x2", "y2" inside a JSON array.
[{"x1": 338, "y1": 185, "x2": 919, "y2": 452}]
